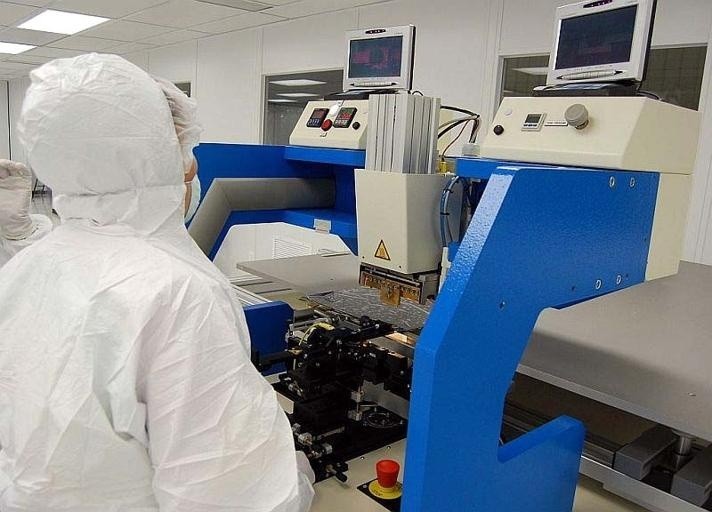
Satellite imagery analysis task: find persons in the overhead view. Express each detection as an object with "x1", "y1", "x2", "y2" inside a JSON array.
[
  {"x1": 0, "y1": 159, "x2": 53, "y2": 268},
  {"x1": 0, "y1": 52, "x2": 316, "y2": 512}
]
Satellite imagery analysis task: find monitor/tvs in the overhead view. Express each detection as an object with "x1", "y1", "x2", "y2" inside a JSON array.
[
  {"x1": 544, "y1": 0, "x2": 658, "y2": 84},
  {"x1": 343, "y1": 23, "x2": 417, "y2": 91}
]
